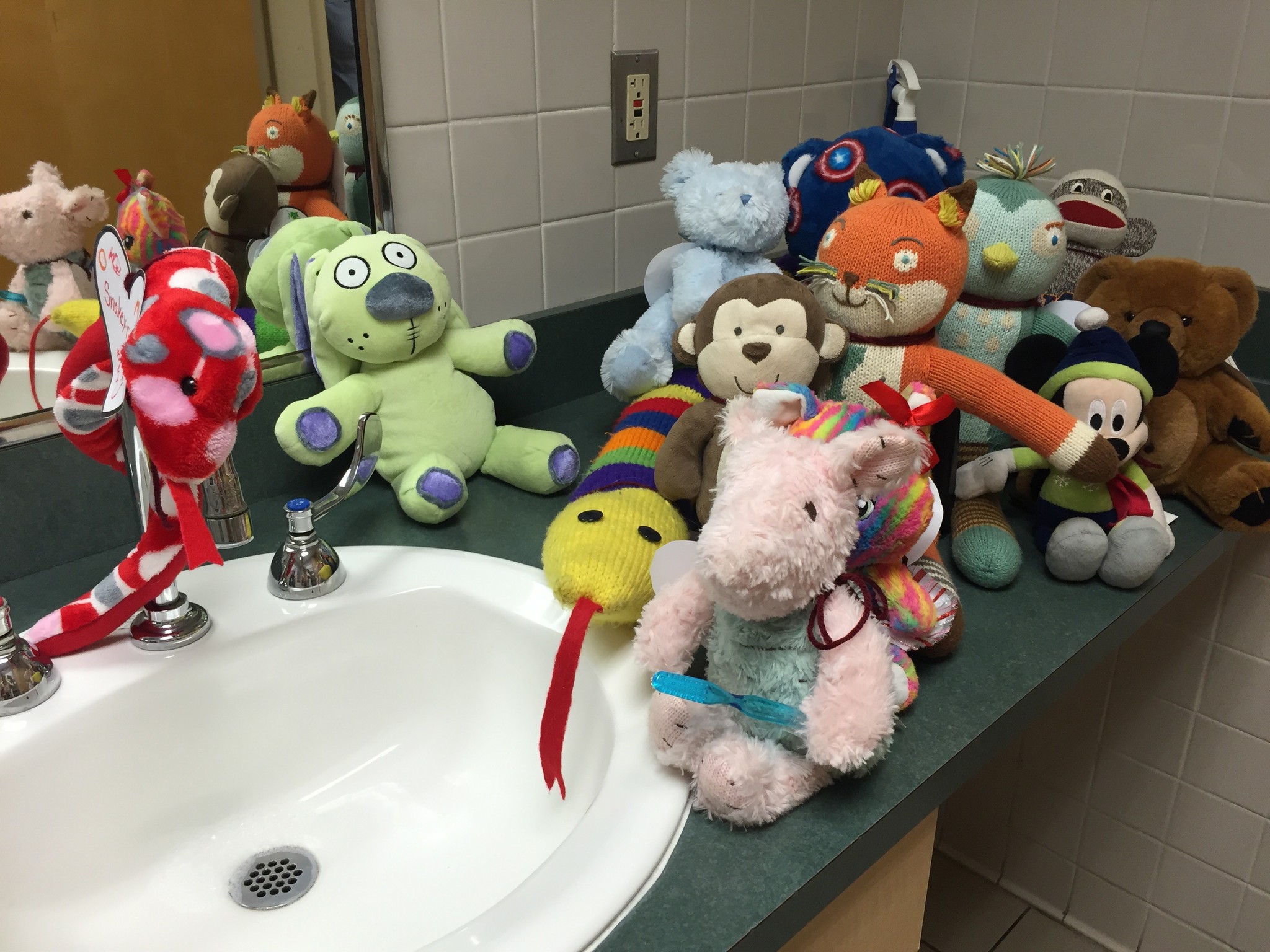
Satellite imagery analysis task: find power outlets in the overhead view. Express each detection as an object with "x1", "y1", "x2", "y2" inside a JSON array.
[{"x1": 611, "y1": 48, "x2": 658, "y2": 166}]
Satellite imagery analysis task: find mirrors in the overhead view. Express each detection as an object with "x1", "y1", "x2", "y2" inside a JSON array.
[{"x1": 0, "y1": 0, "x2": 394, "y2": 446}]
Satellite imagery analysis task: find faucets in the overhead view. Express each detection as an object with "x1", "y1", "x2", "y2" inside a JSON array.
[{"x1": 114, "y1": 393, "x2": 255, "y2": 651}]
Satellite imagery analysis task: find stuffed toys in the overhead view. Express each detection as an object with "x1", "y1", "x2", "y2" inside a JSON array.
[
  {"x1": 190, "y1": 146, "x2": 278, "y2": 308},
  {"x1": 16, "y1": 290, "x2": 264, "y2": 656},
  {"x1": 0, "y1": 161, "x2": 111, "y2": 352},
  {"x1": 238, "y1": 85, "x2": 349, "y2": 224},
  {"x1": 633, "y1": 390, "x2": 929, "y2": 826},
  {"x1": 245, "y1": 218, "x2": 372, "y2": 361},
  {"x1": 270, "y1": 231, "x2": 582, "y2": 526},
  {"x1": 116, "y1": 169, "x2": 190, "y2": 272},
  {"x1": 328, "y1": 95, "x2": 371, "y2": 227},
  {"x1": 29, "y1": 245, "x2": 240, "y2": 401},
  {"x1": 540, "y1": 126, "x2": 1269, "y2": 719}
]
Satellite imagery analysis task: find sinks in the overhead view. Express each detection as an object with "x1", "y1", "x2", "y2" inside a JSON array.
[{"x1": 0, "y1": 536, "x2": 687, "y2": 952}]
